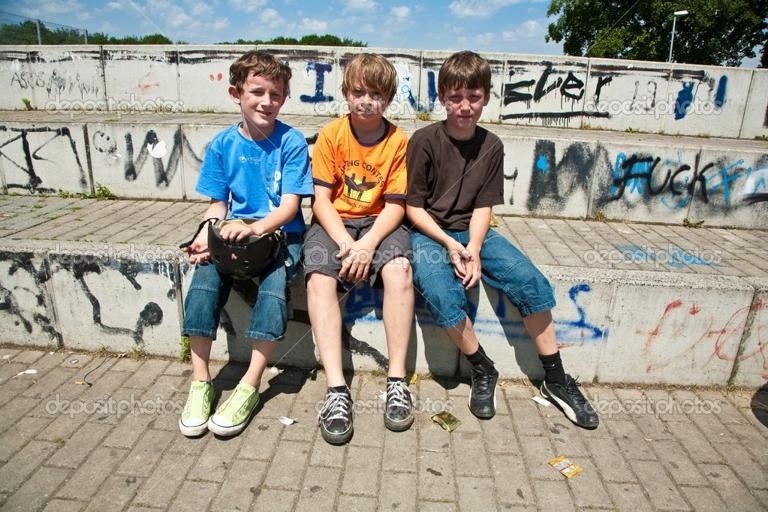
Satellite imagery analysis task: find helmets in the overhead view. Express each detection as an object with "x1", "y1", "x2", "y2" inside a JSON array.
[{"x1": 179, "y1": 217, "x2": 287, "y2": 281}]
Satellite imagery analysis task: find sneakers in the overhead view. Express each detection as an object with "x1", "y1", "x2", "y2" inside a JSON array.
[
  {"x1": 316, "y1": 385, "x2": 353, "y2": 445},
  {"x1": 179, "y1": 381, "x2": 260, "y2": 437},
  {"x1": 467, "y1": 364, "x2": 498, "y2": 419},
  {"x1": 540, "y1": 374, "x2": 599, "y2": 428},
  {"x1": 384, "y1": 377, "x2": 414, "y2": 432}
]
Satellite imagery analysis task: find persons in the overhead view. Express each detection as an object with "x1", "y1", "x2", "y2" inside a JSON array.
[
  {"x1": 304, "y1": 51, "x2": 410, "y2": 446},
  {"x1": 176, "y1": 50, "x2": 314, "y2": 436},
  {"x1": 405, "y1": 50, "x2": 600, "y2": 431}
]
